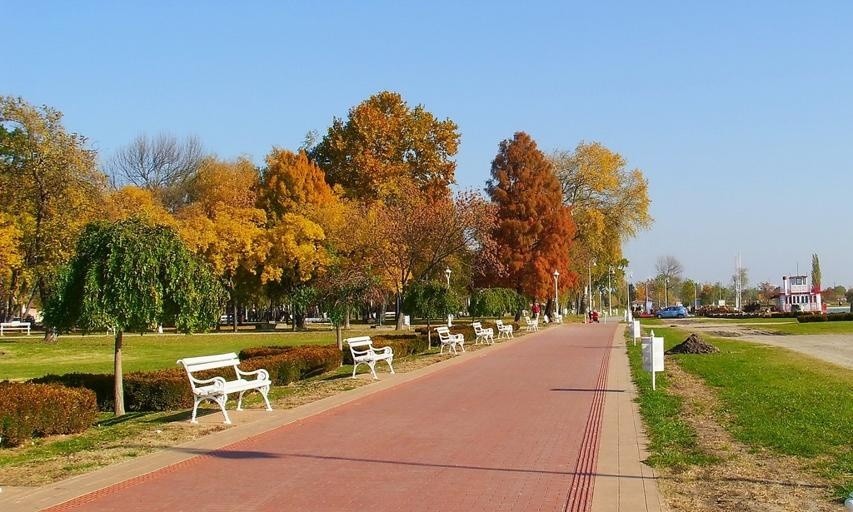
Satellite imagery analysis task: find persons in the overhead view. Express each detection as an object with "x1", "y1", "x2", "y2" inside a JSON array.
[
  {"x1": 588, "y1": 310, "x2": 593, "y2": 320},
  {"x1": 531, "y1": 301, "x2": 541, "y2": 321}
]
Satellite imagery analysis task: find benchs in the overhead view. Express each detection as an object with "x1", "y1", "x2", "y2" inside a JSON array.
[
  {"x1": 0, "y1": 323, "x2": 31, "y2": 335},
  {"x1": 176, "y1": 352, "x2": 272, "y2": 424},
  {"x1": 434, "y1": 315, "x2": 539, "y2": 357}
]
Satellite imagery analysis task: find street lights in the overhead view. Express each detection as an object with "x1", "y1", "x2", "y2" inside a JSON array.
[
  {"x1": 644, "y1": 277, "x2": 654, "y2": 311},
  {"x1": 589, "y1": 257, "x2": 598, "y2": 312},
  {"x1": 627, "y1": 271, "x2": 633, "y2": 314},
  {"x1": 553, "y1": 268, "x2": 562, "y2": 324},
  {"x1": 444, "y1": 264, "x2": 456, "y2": 330},
  {"x1": 666, "y1": 278, "x2": 670, "y2": 307},
  {"x1": 694, "y1": 281, "x2": 700, "y2": 310},
  {"x1": 608, "y1": 266, "x2": 617, "y2": 317}
]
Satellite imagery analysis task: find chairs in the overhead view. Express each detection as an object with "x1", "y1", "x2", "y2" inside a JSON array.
[{"x1": 347, "y1": 336, "x2": 395, "y2": 380}]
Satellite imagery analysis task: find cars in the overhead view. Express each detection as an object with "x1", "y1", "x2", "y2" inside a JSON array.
[
  {"x1": 634, "y1": 311, "x2": 654, "y2": 318},
  {"x1": 656, "y1": 306, "x2": 689, "y2": 320}
]
